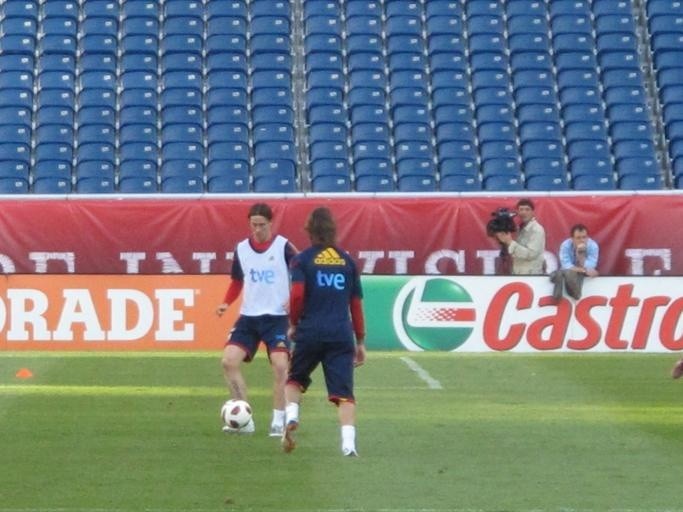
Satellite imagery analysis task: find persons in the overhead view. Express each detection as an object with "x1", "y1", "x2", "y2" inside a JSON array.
[
  {"x1": 280, "y1": 207, "x2": 366, "y2": 458},
  {"x1": 496, "y1": 199, "x2": 546, "y2": 274},
  {"x1": 215, "y1": 204, "x2": 301, "y2": 438},
  {"x1": 558, "y1": 223, "x2": 599, "y2": 276}
]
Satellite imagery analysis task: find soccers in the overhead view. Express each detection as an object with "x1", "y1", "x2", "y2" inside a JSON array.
[{"x1": 220, "y1": 398, "x2": 253, "y2": 428}]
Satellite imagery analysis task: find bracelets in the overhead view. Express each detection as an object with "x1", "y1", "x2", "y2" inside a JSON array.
[
  {"x1": 582, "y1": 267, "x2": 587, "y2": 272},
  {"x1": 355, "y1": 337, "x2": 364, "y2": 346}
]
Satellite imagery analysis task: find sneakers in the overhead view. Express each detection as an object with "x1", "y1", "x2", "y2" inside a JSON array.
[
  {"x1": 341, "y1": 446, "x2": 357, "y2": 457},
  {"x1": 268, "y1": 418, "x2": 301, "y2": 453},
  {"x1": 221, "y1": 419, "x2": 256, "y2": 433}
]
